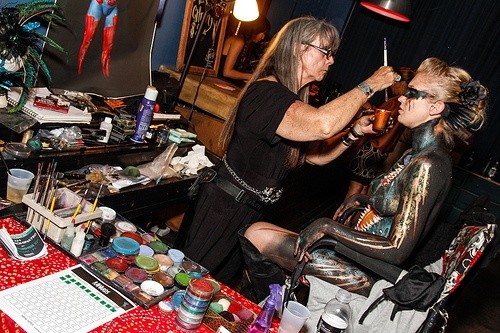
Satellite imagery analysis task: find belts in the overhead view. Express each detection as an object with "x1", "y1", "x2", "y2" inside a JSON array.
[{"x1": 213, "y1": 175, "x2": 267, "y2": 213}]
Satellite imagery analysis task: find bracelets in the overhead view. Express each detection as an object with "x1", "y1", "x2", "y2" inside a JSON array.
[
  {"x1": 357, "y1": 81, "x2": 374, "y2": 97},
  {"x1": 350, "y1": 126, "x2": 364, "y2": 137},
  {"x1": 346, "y1": 134, "x2": 355, "y2": 142},
  {"x1": 341, "y1": 136, "x2": 350, "y2": 147}
]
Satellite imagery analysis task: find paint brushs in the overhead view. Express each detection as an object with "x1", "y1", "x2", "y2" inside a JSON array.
[
  {"x1": 383, "y1": 37, "x2": 389, "y2": 102},
  {"x1": 70, "y1": 188, "x2": 88, "y2": 219},
  {"x1": 25, "y1": 160, "x2": 59, "y2": 242},
  {"x1": 84, "y1": 183, "x2": 106, "y2": 233}
]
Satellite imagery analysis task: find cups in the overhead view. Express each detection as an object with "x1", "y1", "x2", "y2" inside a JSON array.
[
  {"x1": 372, "y1": 108, "x2": 391, "y2": 131},
  {"x1": 278, "y1": 301, "x2": 311, "y2": 333},
  {"x1": 175, "y1": 278, "x2": 214, "y2": 331},
  {"x1": 7, "y1": 169, "x2": 34, "y2": 204}
]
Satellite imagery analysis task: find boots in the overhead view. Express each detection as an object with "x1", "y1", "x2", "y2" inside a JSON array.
[{"x1": 237, "y1": 224, "x2": 290, "y2": 314}]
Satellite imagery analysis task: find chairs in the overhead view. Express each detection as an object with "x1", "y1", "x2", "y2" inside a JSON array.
[{"x1": 282, "y1": 199, "x2": 498, "y2": 333}]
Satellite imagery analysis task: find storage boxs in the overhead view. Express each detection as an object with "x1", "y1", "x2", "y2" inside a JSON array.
[{"x1": 22, "y1": 188, "x2": 102, "y2": 242}]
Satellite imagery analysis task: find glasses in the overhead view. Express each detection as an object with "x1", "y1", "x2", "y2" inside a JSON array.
[{"x1": 300, "y1": 41, "x2": 336, "y2": 60}]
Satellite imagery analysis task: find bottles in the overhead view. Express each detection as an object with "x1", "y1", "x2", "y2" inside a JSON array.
[
  {"x1": 0, "y1": 142, "x2": 31, "y2": 199},
  {"x1": 314, "y1": 288, "x2": 352, "y2": 333},
  {"x1": 97, "y1": 117, "x2": 113, "y2": 143},
  {"x1": 154, "y1": 118, "x2": 171, "y2": 146},
  {"x1": 129, "y1": 86, "x2": 158, "y2": 144}
]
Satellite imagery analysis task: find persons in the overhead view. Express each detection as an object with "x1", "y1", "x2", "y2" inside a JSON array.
[
  {"x1": 176, "y1": 16, "x2": 396, "y2": 289},
  {"x1": 217, "y1": 15, "x2": 272, "y2": 84},
  {"x1": 238, "y1": 58, "x2": 488, "y2": 305}
]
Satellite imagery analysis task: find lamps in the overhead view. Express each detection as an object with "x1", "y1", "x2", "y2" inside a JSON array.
[{"x1": 360, "y1": 0, "x2": 411, "y2": 22}]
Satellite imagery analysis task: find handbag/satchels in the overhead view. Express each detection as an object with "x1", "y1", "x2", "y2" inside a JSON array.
[{"x1": 383, "y1": 269, "x2": 446, "y2": 312}]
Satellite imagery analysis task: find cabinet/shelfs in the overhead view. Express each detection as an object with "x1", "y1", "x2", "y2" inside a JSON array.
[
  {"x1": 437, "y1": 167, "x2": 500, "y2": 225},
  {"x1": 0, "y1": 124, "x2": 281, "y2": 333}
]
